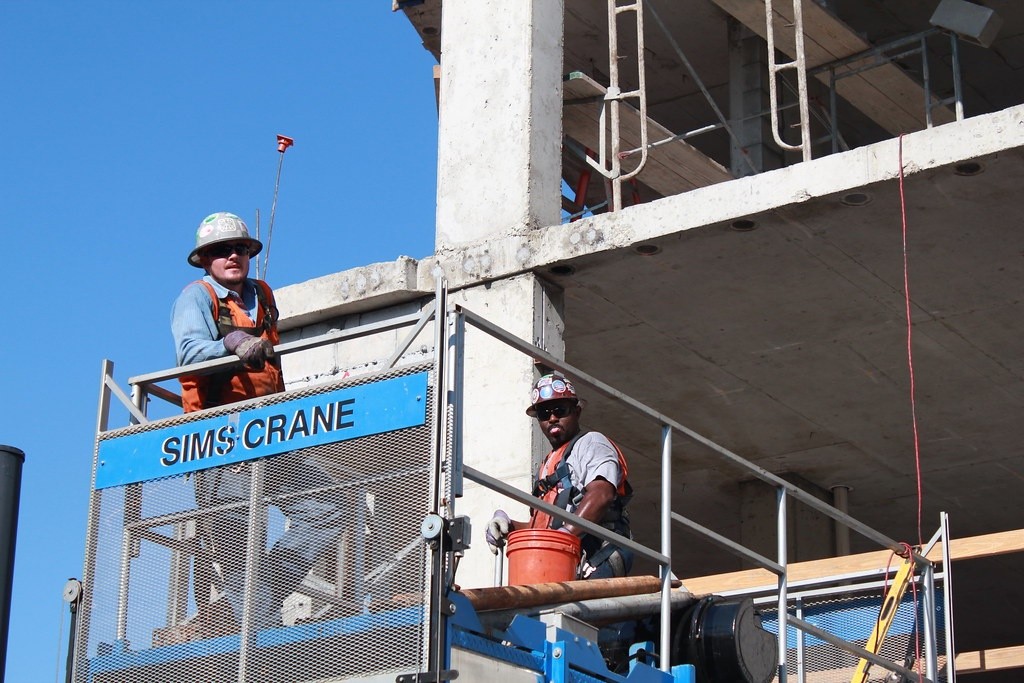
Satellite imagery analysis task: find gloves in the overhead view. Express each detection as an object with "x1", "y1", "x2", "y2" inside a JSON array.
[
  {"x1": 224, "y1": 330, "x2": 275, "y2": 370},
  {"x1": 485, "y1": 508, "x2": 512, "y2": 556}
]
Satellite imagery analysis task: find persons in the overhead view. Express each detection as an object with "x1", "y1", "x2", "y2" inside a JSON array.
[
  {"x1": 484, "y1": 369, "x2": 637, "y2": 584},
  {"x1": 170, "y1": 210, "x2": 348, "y2": 635}
]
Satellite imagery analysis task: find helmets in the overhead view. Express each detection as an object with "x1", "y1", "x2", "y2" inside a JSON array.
[
  {"x1": 188, "y1": 211, "x2": 263, "y2": 268},
  {"x1": 525, "y1": 374, "x2": 577, "y2": 417}
]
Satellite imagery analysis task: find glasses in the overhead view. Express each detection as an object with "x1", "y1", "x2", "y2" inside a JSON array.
[
  {"x1": 536, "y1": 405, "x2": 577, "y2": 421},
  {"x1": 204, "y1": 243, "x2": 250, "y2": 258}
]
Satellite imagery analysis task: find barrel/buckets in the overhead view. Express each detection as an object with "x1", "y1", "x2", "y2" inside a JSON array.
[{"x1": 506, "y1": 529, "x2": 580, "y2": 587}]
